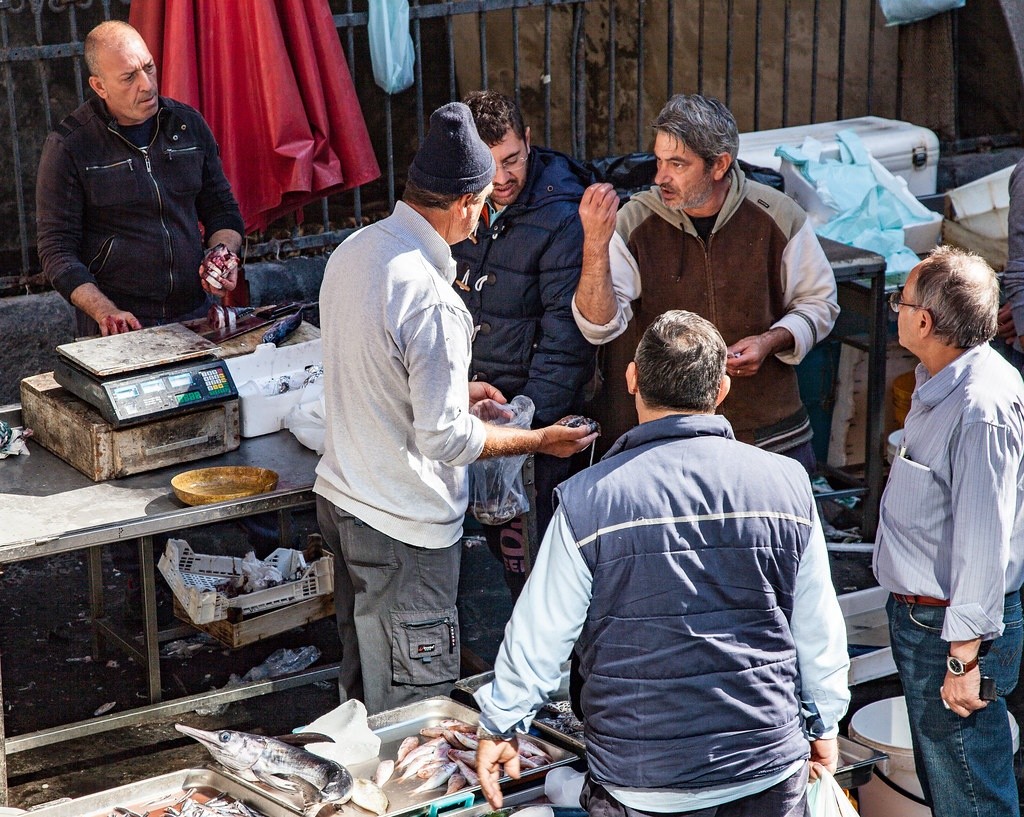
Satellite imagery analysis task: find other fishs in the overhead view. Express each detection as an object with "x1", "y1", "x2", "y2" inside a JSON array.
[
  {"x1": 174, "y1": 721, "x2": 354, "y2": 817},
  {"x1": 107, "y1": 785, "x2": 267, "y2": 817},
  {"x1": 262, "y1": 305, "x2": 304, "y2": 345},
  {"x1": 351, "y1": 701, "x2": 555, "y2": 817}
]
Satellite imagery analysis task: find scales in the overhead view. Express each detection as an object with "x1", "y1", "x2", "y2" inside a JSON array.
[{"x1": 53, "y1": 320, "x2": 240, "y2": 430}]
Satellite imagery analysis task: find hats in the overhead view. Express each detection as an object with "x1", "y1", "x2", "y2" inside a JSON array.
[{"x1": 408, "y1": 102, "x2": 496, "y2": 196}]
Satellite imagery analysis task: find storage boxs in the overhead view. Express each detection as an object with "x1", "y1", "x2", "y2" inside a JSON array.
[
  {"x1": 943, "y1": 220, "x2": 1010, "y2": 271},
  {"x1": 826, "y1": 331, "x2": 919, "y2": 467},
  {"x1": 224, "y1": 338, "x2": 323, "y2": 437},
  {"x1": 780, "y1": 144, "x2": 943, "y2": 253},
  {"x1": 736, "y1": 116, "x2": 940, "y2": 196},
  {"x1": 943, "y1": 163, "x2": 1018, "y2": 240},
  {"x1": 173, "y1": 593, "x2": 337, "y2": 648}
]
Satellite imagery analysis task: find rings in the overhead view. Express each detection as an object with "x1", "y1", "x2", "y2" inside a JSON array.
[{"x1": 737, "y1": 370, "x2": 740, "y2": 376}]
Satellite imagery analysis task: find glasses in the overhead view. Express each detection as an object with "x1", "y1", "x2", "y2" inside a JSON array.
[
  {"x1": 495, "y1": 144, "x2": 529, "y2": 172},
  {"x1": 888, "y1": 291, "x2": 923, "y2": 313}
]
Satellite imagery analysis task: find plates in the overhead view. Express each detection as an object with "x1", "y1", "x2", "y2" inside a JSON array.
[{"x1": 171, "y1": 466, "x2": 278, "y2": 506}]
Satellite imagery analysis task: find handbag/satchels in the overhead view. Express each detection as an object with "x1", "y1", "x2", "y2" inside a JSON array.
[{"x1": 805, "y1": 760, "x2": 860, "y2": 817}]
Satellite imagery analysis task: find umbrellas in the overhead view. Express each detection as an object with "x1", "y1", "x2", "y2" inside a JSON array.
[{"x1": 129, "y1": 0, "x2": 381, "y2": 235}]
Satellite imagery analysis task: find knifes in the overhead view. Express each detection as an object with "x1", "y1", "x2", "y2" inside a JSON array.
[{"x1": 201, "y1": 301, "x2": 301, "y2": 344}]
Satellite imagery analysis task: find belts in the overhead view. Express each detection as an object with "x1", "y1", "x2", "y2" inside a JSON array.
[{"x1": 891, "y1": 591, "x2": 952, "y2": 607}]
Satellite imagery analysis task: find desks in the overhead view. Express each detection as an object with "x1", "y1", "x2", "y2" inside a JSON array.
[
  {"x1": 812, "y1": 234, "x2": 887, "y2": 541},
  {"x1": 0, "y1": 403, "x2": 341, "y2": 808}
]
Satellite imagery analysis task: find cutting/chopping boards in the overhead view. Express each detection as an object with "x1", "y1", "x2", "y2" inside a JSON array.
[{"x1": 181, "y1": 304, "x2": 321, "y2": 359}]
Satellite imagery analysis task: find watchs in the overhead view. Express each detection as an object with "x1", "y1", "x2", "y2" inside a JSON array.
[
  {"x1": 943, "y1": 654, "x2": 980, "y2": 677},
  {"x1": 476, "y1": 726, "x2": 504, "y2": 741}
]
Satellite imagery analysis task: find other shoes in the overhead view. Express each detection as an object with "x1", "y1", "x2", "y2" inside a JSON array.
[{"x1": 126, "y1": 572, "x2": 165, "y2": 607}]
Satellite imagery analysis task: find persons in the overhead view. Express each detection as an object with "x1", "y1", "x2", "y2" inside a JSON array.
[
  {"x1": 1004, "y1": 156, "x2": 1024, "y2": 379},
  {"x1": 571, "y1": 92, "x2": 843, "y2": 502},
  {"x1": 471, "y1": 308, "x2": 852, "y2": 817},
  {"x1": 36, "y1": 20, "x2": 245, "y2": 338},
  {"x1": 872, "y1": 245, "x2": 1024, "y2": 817},
  {"x1": 310, "y1": 89, "x2": 603, "y2": 718}
]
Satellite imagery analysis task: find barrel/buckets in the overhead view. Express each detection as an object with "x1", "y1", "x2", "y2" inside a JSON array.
[{"x1": 848, "y1": 695, "x2": 1019, "y2": 817}]
